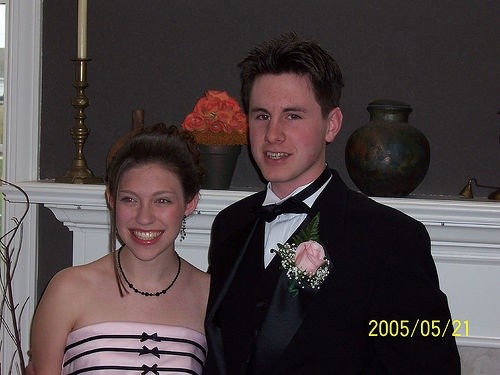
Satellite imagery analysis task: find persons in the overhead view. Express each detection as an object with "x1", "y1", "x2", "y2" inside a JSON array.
[
  {"x1": 202, "y1": 34, "x2": 462, "y2": 375},
  {"x1": 31, "y1": 124, "x2": 210, "y2": 375}
]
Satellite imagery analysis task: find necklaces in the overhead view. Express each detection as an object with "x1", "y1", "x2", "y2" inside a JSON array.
[{"x1": 118, "y1": 244, "x2": 181, "y2": 296}]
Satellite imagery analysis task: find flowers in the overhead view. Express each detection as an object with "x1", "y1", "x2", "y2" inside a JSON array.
[
  {"x1": 271, "y1": 209, "x2": 332, "y2": 290},
  {"x1": 182, "y1": 90, "x2": 250, "y2": 146}
]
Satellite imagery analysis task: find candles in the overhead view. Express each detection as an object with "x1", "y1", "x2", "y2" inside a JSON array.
[{"x1": 75, "y1": 0, "x2": 88, "y2": 59}]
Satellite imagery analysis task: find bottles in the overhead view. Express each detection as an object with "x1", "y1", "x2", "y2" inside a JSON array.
[{"x1": 344, "y1": 101, "x2": 430, "y2": 198}]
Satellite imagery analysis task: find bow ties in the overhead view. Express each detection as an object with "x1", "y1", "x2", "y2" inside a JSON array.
[{"x1": 256, "y1": 162, "x2": 332, "y2": 224}]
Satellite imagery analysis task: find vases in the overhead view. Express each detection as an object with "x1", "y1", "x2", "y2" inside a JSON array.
[
  {"x1": 345, "y1": 105, "x2": 431, "y2": 198},
  {"x1": 194, "y1": 145, "x2": 242, "y2": 190}
]
